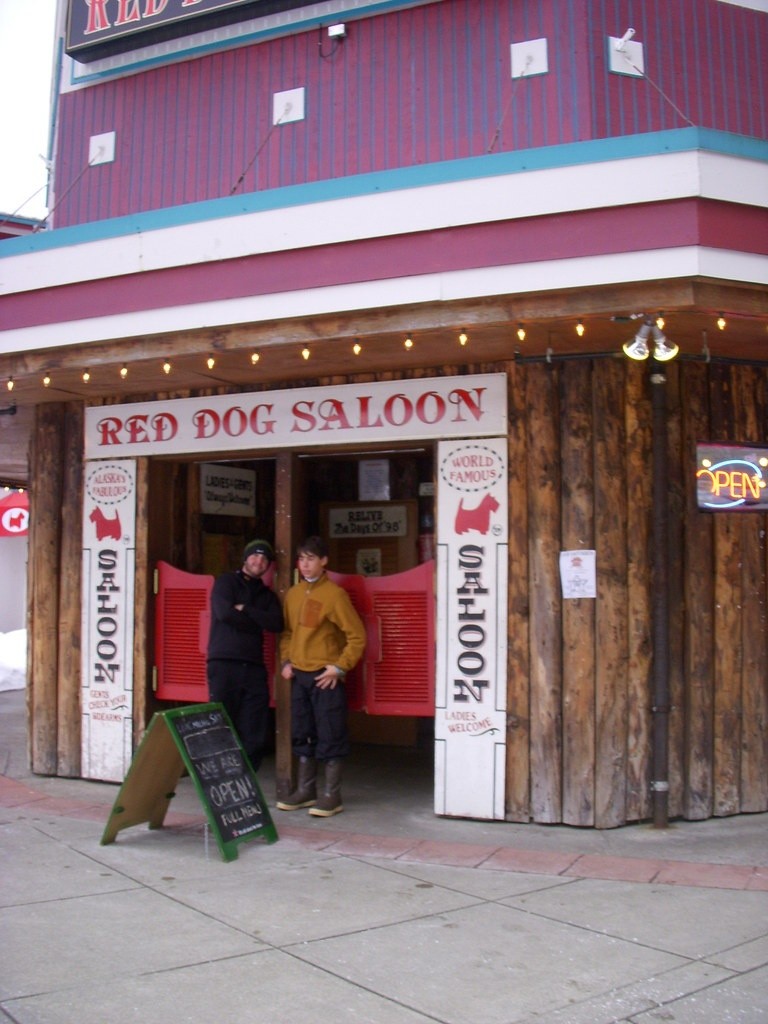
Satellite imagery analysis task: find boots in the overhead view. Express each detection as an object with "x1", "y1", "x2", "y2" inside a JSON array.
[
  {"x1": 276, "y1": 764, "x2": 317, "y2": 811},
  {"x1": 309, "y1": 763, "x2": 344, "y2": 816}
]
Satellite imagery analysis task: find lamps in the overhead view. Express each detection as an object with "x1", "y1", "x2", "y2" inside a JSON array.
[
  {"x1": 651, "y1": 321, "x2": 679, "y2": 362},
  {"x1": 622, "y1": 318, "x2": 653, "y2": 361}
]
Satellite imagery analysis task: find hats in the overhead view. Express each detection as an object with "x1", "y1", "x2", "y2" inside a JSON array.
[{"x1": 243, "y1": 539, "x2": 275, "y2": 564}]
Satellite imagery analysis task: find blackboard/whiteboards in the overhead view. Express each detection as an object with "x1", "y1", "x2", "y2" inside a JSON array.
[{"x1": 160, "y1": 703, "x2": 279, "y2": 863}]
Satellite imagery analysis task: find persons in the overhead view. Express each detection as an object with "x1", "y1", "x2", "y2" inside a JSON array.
[
  {"x1": 205, "y1": 539, "x2": 284, "y2": 779},
  {"x1": 276, "y1": 535, "x2": 367, "y2": 817}
]
